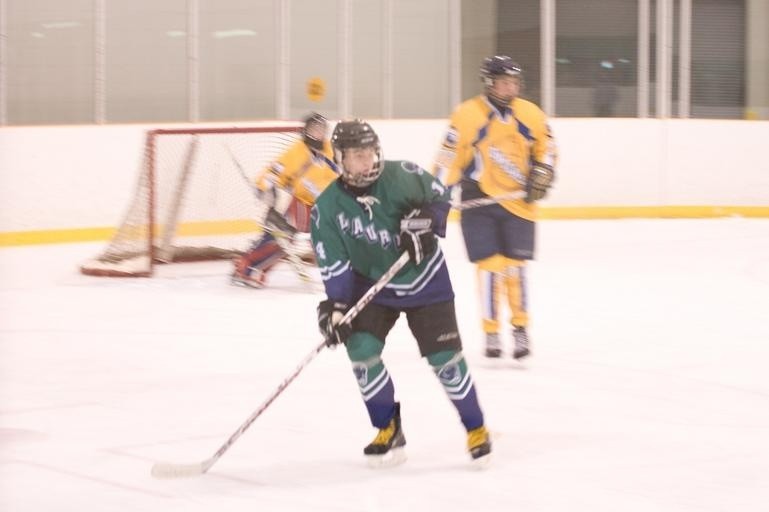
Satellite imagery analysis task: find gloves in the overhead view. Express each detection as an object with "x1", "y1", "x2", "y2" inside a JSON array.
[
  {"x1": 524, "y1": 161, "x2": 554, "y2": 203},
  {"x1": 399, "y1": 208, "x2": 440, "y2": 268},
  {"x1": 317, "y1": 298, "x2": 355, "y2": 349}
]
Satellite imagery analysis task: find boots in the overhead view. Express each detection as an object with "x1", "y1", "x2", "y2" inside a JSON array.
[{"x1": 363, "y1": 401, "x2": 406, "y2": 456}]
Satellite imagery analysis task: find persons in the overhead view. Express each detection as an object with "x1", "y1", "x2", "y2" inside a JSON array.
[
  {"x1": 230, "y1": 111, "x2": 344, "y2": 289},
  {"x1": 306, "y1": 116, "x2": 493, "y2": 461},
  {"x1": 429, "y1": 55, "x2": 558, "y2": 362}
]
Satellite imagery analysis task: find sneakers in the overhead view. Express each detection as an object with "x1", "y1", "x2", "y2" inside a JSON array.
[
  {"x1": 513, "y1": 327, "x2": 531, "y2": 359},
  {"x1": 486, "y1": 332, "x2": 502, "y2": 359},
  {"x1": 465, "y1": 423, "x2": 493, "y2": 459}
]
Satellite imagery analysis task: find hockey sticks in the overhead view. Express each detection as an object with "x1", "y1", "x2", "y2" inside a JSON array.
[
  {"x1": 151, "y1": 249, "x2": 410, "y2": 485},
  {"x1": 228, "y1": 146, "x2": 322, "y2": 289}
]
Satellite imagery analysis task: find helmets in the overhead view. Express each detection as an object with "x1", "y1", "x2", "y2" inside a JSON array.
[
  {"x1": 331, "y1": 117, "x2": 386, "y2": 188},
  {"x1": 303, "y1": 112, "x2": 330, "y2": 150},
  {"x1": 478, "y1": 55, "x2": 528, "y2": 93}
]
